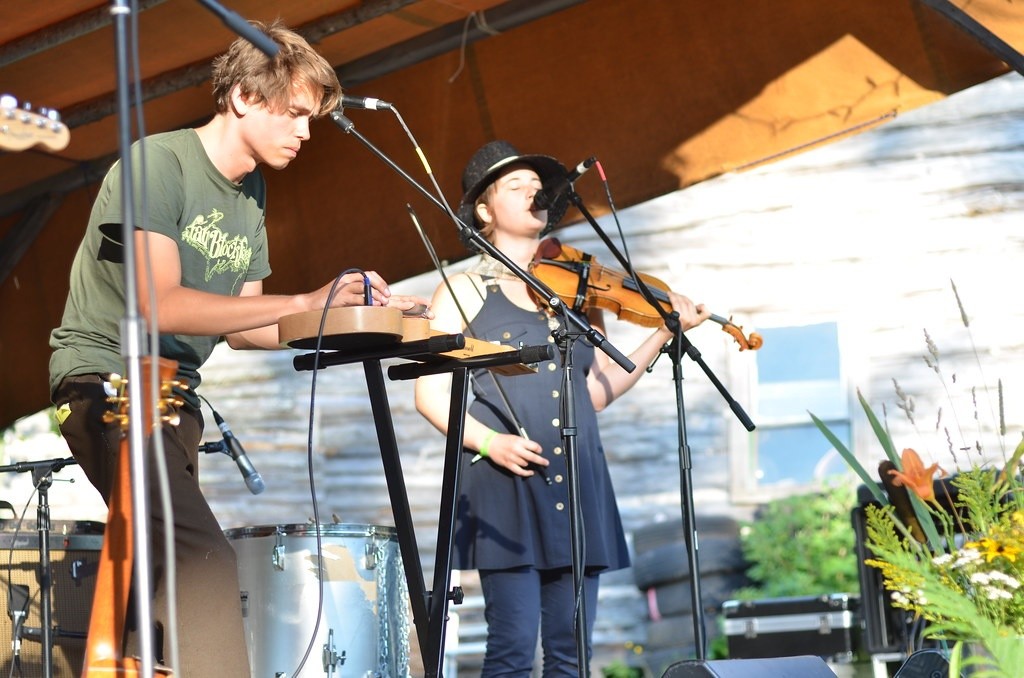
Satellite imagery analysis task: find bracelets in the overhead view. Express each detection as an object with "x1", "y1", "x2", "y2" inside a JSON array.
[{"x1": 470, "y1": 430, "x2": 499, "y2": 465}]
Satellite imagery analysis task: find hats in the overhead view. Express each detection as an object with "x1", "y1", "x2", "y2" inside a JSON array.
[{"x1": 457, "y1": 142, "x2": 572, "y2": 239}]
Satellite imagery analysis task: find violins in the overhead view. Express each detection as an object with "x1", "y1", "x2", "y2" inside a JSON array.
[{"x1": 528, "y1": 237, "x2": 764, "y2": 353}]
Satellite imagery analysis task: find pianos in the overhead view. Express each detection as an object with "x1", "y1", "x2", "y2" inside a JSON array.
[{"x1": 274, "y1": 307, "x2": 543, "y2": 379}]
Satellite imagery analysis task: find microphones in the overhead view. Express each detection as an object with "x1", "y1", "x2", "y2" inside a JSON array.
[
  {"x1": 337, "y1": 92, "x2": 394, "y2": 110},
  {"x1": 212, "y1": 410, "x2": 265, "y2": 496},
  {"x1": 533, "y1": 157, "x2": 594, "y2": 210}
]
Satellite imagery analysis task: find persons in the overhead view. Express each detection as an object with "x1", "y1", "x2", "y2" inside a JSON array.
[
  {"x1": 413, "y1": 143, "x2": 712, "y2": 678},
  {"x1": 45, "y1": 19, "x2": 345, "y2": 678}
]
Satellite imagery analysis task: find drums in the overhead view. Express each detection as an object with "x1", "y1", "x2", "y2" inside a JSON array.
[{"x1": 209, "y1": 519, "x2": 424, "y2": 678}]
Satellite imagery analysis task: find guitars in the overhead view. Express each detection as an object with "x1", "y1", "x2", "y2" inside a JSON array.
[{"x1": 0, "y1": 90, "x2": 71, "y2": 155}]
